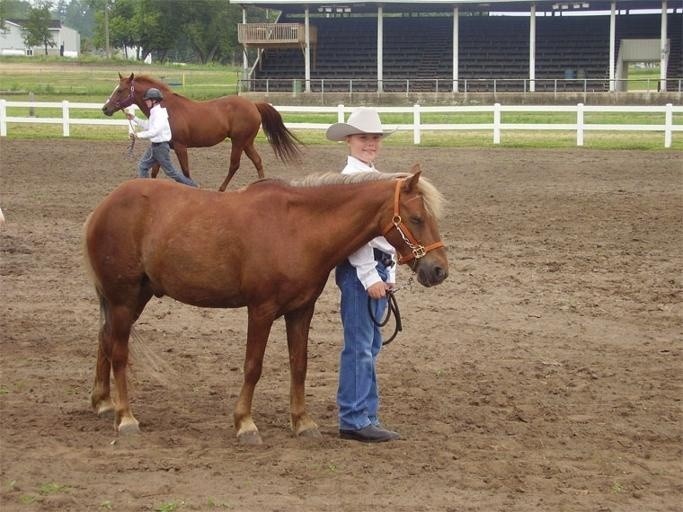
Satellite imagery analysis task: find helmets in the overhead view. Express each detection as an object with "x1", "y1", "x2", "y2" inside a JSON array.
[{"x1": 143, "y1": 88, "x2": 164, "y2": 101}]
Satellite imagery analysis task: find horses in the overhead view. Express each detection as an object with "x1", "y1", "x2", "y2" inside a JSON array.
[
  {"x1": 80, "y1": 165, "x2": 449, "y2": 449},
  {"x1": 102, "y1": 72, "x2": 308, "y2": 192}
]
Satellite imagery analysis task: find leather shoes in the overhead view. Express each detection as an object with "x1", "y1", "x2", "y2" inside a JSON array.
[{"x1": 339, "y1": 424, "x2": 401, "y2": 443}]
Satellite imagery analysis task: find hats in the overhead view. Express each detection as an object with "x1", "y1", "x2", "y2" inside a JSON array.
[{"x1": 326, "y1": 109, "x2": 396, "y2": 141}]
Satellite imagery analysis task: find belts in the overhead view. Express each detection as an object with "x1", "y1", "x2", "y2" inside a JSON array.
[{"x1": 374, "y1": 249, "x2": 394, "y2": 267}]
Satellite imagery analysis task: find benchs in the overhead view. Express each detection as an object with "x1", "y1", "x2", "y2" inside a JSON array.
[{"x1": 249, "y1": 37, "x2": 609, "y2": 92}]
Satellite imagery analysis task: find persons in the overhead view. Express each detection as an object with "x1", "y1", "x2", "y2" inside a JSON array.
[
  {"x1": 127, "y1": 88, "x2": 200, "y2": 189},
  {"x1": 326, "y1": 107, "x2": 401, "y2": 443}
]
